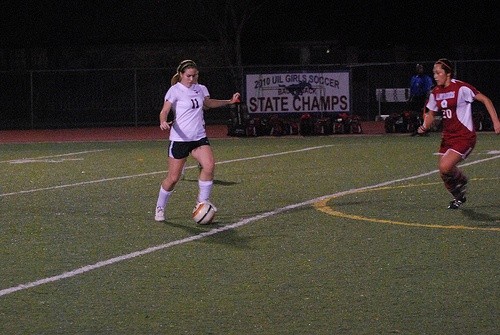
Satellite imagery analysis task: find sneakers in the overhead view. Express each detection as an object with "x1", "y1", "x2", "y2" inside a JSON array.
[
  {"x1": 458, "y1": 177, "x2": 467, "y2": 194},
  {"x1": 448, "y1": 197, "x2": 466, "y2": 209},
  {"x1": 154, "y1": 206, "x2": 166, "y2": 222},
  {"x1": 197, "y1": 200, "x2": 217, "y2": 212}
]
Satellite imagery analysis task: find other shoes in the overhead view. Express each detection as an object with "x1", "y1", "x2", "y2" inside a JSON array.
[
  {"x1": 409, "y1": 133, "x2": 420, "y2": 138},
  {"x1": 425, "y1": 130, "x2": 431, "y2": 137}
]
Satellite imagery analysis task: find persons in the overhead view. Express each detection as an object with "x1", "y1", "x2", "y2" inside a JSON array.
[
  {"x1": 408, "y1": 63, "x2": 434, "y2": 137},
  {"x1": 417, "y1": 59, "x2": 500, "y2": 209},
  {"x1": 176, "y1": 66, "x2": 210, "y2": 180},
  {"x1": 154, "y1": 59, "x2": 241, "y2": 222}
]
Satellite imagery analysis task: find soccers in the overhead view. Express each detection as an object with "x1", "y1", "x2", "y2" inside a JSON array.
[{"x1": 192, "y1": 201, "x2": 215, "y2": 225}]
